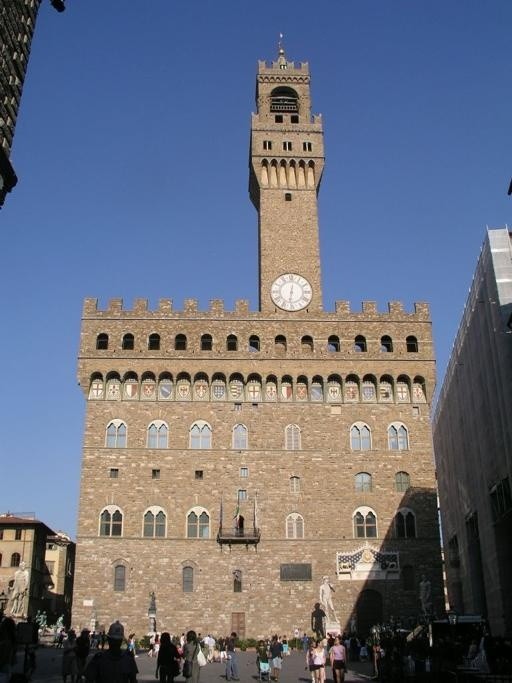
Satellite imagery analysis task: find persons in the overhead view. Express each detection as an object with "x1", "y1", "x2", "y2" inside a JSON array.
[
  {"x1": 319, "y1": 576, "x2": 338, "y2": 622},
  {"x1": 9, "y1": 562, "x2": 29, "y2": 613},
  {"x1": 418, "y1": 575, "x2": 432, "y2": 616},
  {"x1": 149, "y1": 591, "x2": 156, "y2": 609},
  {"x1": 40, "y1": 611, "x2": 48, "y2": 625},
  {"x1": 35, "y1": 610, "x2": 41, "y2": 624}
]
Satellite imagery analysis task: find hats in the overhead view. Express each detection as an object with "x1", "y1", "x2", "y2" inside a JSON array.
[{"x1": 105, "y1": 620, "x2": 125, "y2": 640}]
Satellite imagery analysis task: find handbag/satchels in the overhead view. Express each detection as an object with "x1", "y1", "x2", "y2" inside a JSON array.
[
  {"x1": 183, "y1": 659, "x2": 193, "y2": 678},
  {"x1": 197, "y1": 651, "x2": 207, "y2": 667}
]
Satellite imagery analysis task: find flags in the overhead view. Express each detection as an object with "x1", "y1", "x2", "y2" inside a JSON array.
[{"x1": 232, "y1": 502, "x2": 240, "y2": 530}]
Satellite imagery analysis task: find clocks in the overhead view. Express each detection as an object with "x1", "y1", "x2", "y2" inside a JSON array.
[{"x1": 269, "y1": 271, "x2": 314, "y2": 313}]
